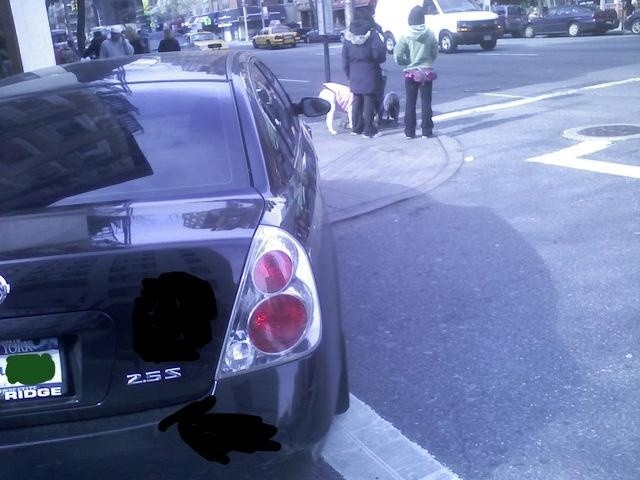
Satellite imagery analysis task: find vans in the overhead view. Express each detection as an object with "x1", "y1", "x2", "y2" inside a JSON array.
[{"x1": 493, "y1": 5, "x2": 528, "y2": 37}]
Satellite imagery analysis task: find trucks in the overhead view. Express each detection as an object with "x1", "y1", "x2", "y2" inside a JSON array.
[{"x1": 373, "y1": 0, "x2": 504, "y2": 56}]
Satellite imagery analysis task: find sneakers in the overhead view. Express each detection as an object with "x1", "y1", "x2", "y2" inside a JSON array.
[
  {"x1": 350, "y1": 129, "x2": 382, "y2": 137},
  {"x1": 403, "y1": 131, "x2": 429, "y2": 138}
]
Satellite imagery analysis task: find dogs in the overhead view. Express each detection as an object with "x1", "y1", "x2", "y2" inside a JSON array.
[
  {"x1": 383, "y1": 91, "x2": 400, "y2": 127},
  {"x1": 319, "y1": 83, "x2": 379, "y2": 135}
]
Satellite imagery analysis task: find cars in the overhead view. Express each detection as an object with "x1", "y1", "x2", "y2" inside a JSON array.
[
  {"x1": 525, "y1": 2, "x2": 622, "y2": 40},
  {"x1": 622, "y1": 8, "x2": 640, "y2": 35},
  {"x1": 0, "y1": 45, "x2": 359, "y2": 480},
  {"x1": 47, "y1": 17, "x2": 347, "y2": 53}
]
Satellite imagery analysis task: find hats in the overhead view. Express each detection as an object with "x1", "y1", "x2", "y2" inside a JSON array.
[
  {"x1": 111, "y1": 25, "x2": 122, "y2": 33},
  {"x1": 408, "y1": 5, "x2": 424, "y2": 25}
]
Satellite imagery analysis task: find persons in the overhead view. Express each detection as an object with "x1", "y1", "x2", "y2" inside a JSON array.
[
  {"x1": 55, "y1": 24, "x2": 181, "y2": 61},
  {"x1": 341, "y1": 8, "x2": 385, "y2": 138},
  {"x1": 363, "y1": 5, "x2": 385, "y2": 124},
  {"x1": 392, "y1": 5, "x2": 439, "y2": 141}
]
